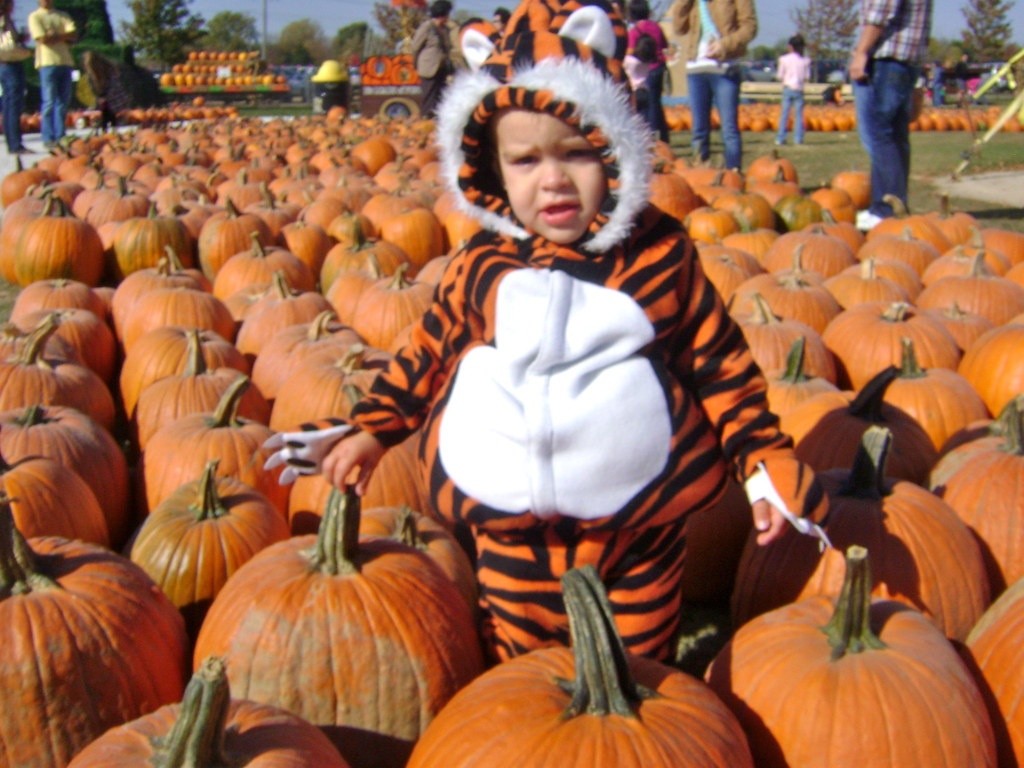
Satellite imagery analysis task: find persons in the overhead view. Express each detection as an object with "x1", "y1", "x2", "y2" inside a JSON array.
[
  {"x1": 773, "y1": 34, "x2": 812, "y2": 145},
  {"x1": 851, "y1": 0, "x2": 934, "y2": 231},
  {"x1": 0, "y1": 0, "x2": 38, "y2": 154},
  {"x1": 311, "y1": 60, "x2": 351, "y2": 117},
  {"x1": 409, "y1": 0, "x2": 670, "y2": 149},
  {"x1": 262, "y1": 1, "x2": 828, "y2": 671},
  {"x1": 668, "y1": 1, "x2": 757, "y2": 173},
  {"x1": 916, "y1": 52, "x2": 976, "y2": 110},
  {"x1": 30, "y1": 0, "x2": 78, "y2": 147}
]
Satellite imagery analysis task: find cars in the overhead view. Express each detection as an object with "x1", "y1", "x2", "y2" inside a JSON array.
[{"x1": 739, "y1": 61, "x2": 775, "y2": 82}]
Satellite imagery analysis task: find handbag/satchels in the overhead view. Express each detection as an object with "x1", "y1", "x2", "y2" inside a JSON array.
[
  {"x1": 629, "y1": 21, "x2": 657, "y2": 64},
  {"x1": 440, "y1": 58, "x2": 455, "y2": 74},
  {"x1": 0, "y1": 30, "x2": 31, "y2": 62},
  {"x1": 684, "y1": 42, "x2": 727, "y2": 74}
]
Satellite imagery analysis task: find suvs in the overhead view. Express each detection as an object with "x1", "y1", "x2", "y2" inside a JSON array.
[
  {"x1": 808, "y1": 61, "x2": 848, "y2": 84},
  {"x1": 965, "y1": 62, "x2": 1011, "y2": 93}
]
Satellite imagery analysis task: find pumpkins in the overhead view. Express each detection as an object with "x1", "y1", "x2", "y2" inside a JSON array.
[
  {"x1": 159, "y1": 50, "x2": 286, "y2": 88},
  {"x1": 0, "y1": 97, "x2": 1024, "y2": 768}
]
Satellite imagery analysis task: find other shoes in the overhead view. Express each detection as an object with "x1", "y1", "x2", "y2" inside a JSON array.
[
  {"x1": 16, "y1": 146, "x2": 33, "y2": 153},
  {"x1": 856, "y1": 210, "x2": 882, "y2": 230}
]
